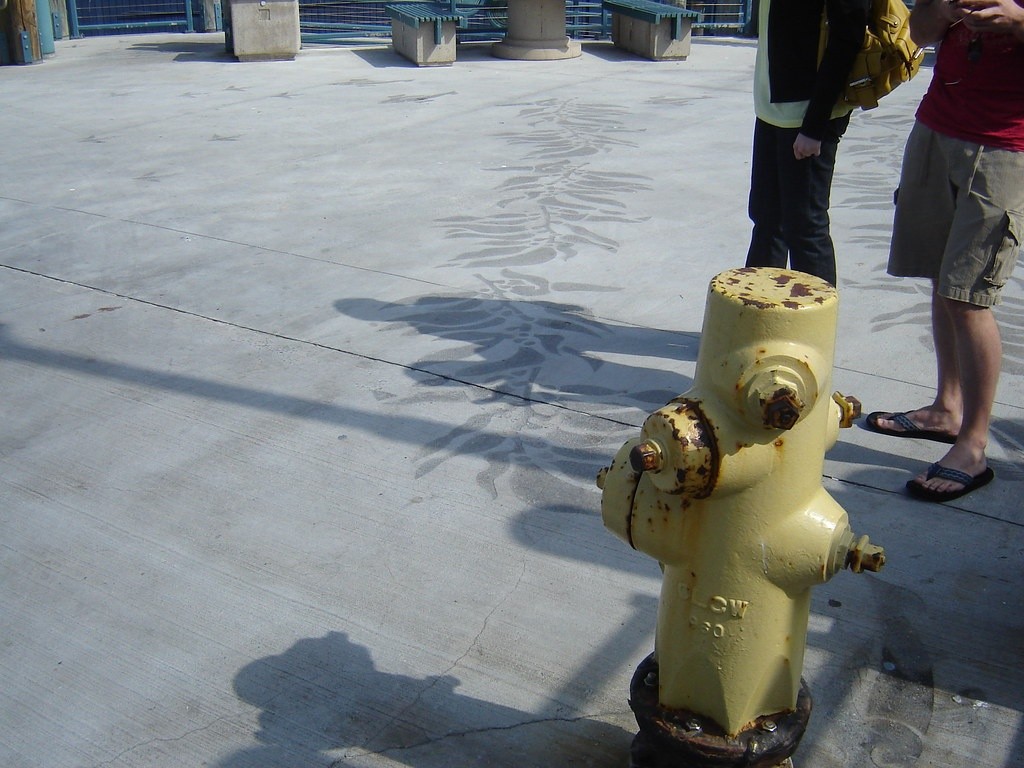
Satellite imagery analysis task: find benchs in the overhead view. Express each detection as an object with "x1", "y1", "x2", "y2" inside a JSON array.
[
  {"x1": 602, "y1": 0, "x2": 702, "y2": 62},
  {"x1": 384, "y1": 3, "x2": 463, "y2": 66}
]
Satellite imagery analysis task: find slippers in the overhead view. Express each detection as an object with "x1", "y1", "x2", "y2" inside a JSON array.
[
  {"x1": 866, "y1": 410, "x2": 958, "y2": 444},
  {"x1": 906, "y1": 461, "x2": 994, "y2": 502}
]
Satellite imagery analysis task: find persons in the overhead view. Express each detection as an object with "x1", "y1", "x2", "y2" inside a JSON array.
[
  {"x1": 865, "y1": 0, "x2": 1024, "y2": 501},
  {"x1": 739, "y1": 0, "x2": 872, "y2": 295}
]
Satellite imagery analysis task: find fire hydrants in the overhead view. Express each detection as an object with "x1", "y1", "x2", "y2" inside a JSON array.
[{"x1": 594, "y1": 263, "x2": 886, "y2": 768}]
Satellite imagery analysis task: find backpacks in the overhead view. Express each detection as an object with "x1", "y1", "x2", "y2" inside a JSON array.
[{"x1": 817, "y1": 0, "x2": 924, "y2": 110}]
